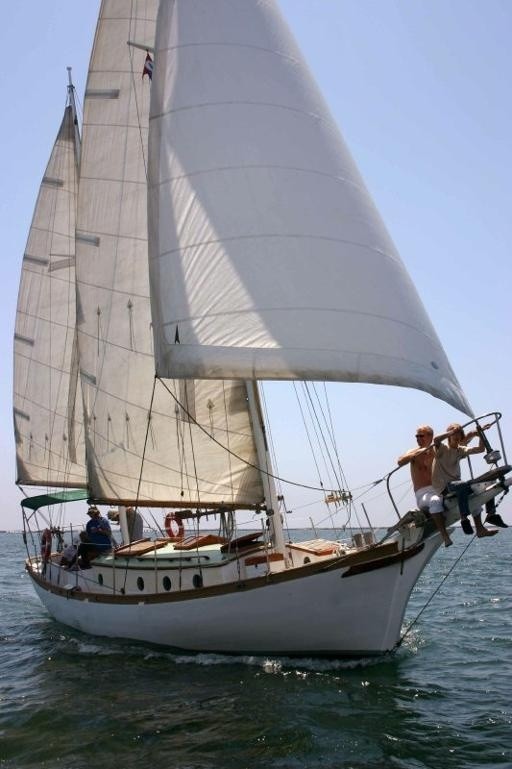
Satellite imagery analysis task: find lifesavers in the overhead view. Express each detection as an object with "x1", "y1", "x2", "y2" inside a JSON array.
[
  {"x1": 165, "y1": 512, "x2": 184, "y2": 541},
  {"x1": 40, "y1": 529, "x2": 52, "y2": 560}
]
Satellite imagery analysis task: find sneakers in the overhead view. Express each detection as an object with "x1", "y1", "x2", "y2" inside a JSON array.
[
  {"x1": 460, "y1": 517, "x2": 473, "y2": 534},
  {"x1": 484, "y1": 514, "x2": 508, "y2": 528}
]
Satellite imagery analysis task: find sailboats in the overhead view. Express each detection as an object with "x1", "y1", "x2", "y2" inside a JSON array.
[{"x1": 11, "y1": 0, "x2": 512, "y2": 661}]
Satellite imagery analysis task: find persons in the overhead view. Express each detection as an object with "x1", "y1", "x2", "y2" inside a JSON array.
[
  {"x1": 77, "y1": 530, "x2": 98, "y2": 566},
  {"x1": 64, "y1": 506, "x2": 112, "y2": 571},
  {"x1": 398, "y1": 425, "x2": 498, "y2": 548},
  {"x1": 432, "y1": 423, "x2": 508, "y2": 536},
  {"x1": 120, "y1": 504, "x2": 144, "y2": 547}
]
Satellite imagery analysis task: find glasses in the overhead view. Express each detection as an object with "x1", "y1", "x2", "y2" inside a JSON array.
[{"x1": 414, "y1": 434, "x2": 430, "y2": 438}]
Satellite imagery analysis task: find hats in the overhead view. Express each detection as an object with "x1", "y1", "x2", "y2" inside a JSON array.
[{"x1": 87, "y1": 507, "x2": 101, "y2": 514}]
